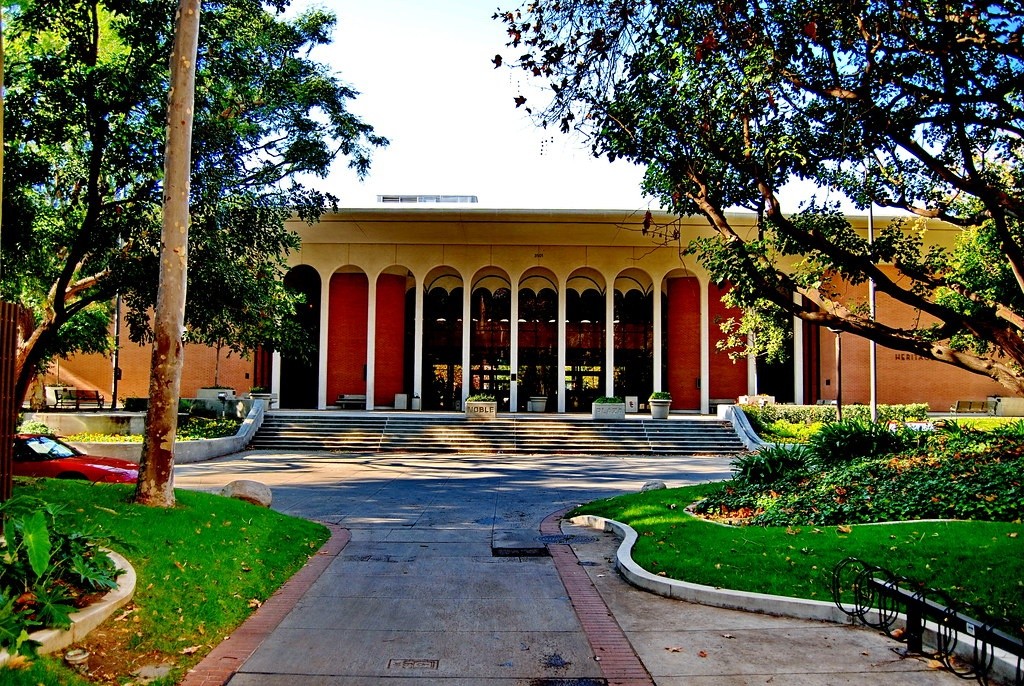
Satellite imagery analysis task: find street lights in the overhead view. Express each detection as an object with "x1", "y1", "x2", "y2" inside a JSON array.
[{"x1": 830, "y1": 85, "x2": 876, "y2": 428}]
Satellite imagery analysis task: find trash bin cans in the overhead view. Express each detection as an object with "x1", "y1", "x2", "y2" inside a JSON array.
[
  {"x1": 394, "y1": 393, "x2": 407, "y2": 410},
  {"x1": 411, "y1": 397, "x2": 422, "y2": 412}
]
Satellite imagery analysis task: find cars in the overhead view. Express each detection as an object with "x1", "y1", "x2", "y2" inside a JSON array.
[{"x1": 9, "y1": 434, "x2": 139, "y2": 485}]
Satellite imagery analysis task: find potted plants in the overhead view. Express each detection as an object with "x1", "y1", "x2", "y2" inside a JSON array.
[
  {"x1": 197, "y1": 387, "x2": 237, "y2": 400},
  {"x1": 46, "y1": 383, "x2": 76, "y2": 403},
  {"x1": 648, "y1": 391, "x2": 673, "y2": 419},
  {"x1": 465, "y1": 393, "x2": 497, "y2": 418},
  {"x1": 591, "y1": 395, "x2": 626, "y2": 419},
  {"x1": 249, "y1": 386, "x2": 272, "y2": 411}
]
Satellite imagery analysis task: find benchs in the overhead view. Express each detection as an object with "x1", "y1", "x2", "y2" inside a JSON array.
[
  {"x1": 710, "y1": 399, "x2": 736, "y2": 413},
  {"x1": 950, "y1": 399, "x2": 995, "y2": 418},
  {"x1": 335, "y1": 394, "x2": 366, "y2": 408},
  {"x1": 53, "y1": 389, "x2": 105, "y2": 409}
]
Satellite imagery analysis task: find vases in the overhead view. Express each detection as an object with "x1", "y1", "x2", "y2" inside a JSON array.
[{"x1": 529, "y1": 396, "x2": 547, "y2": 412}]
